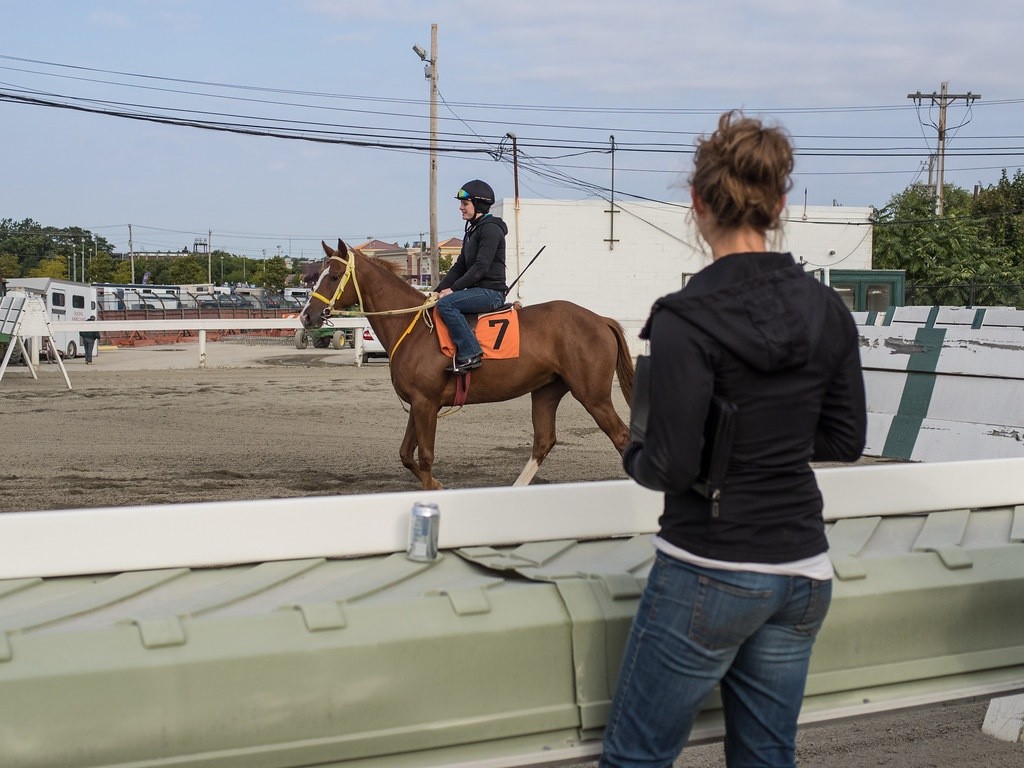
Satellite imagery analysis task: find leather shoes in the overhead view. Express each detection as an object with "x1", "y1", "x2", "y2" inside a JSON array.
[{"x1": 445, "y1": 355, "x2": 483, "y2": 370}]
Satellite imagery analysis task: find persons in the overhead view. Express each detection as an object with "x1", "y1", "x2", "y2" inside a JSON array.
[
  {"x1": 599, "y1": 108, "x2": 867, "y2": 768},
  {"x1": 426, "y1": 179, "x2": 508, "y2": 372},
  {"x1": 79, "y1": 316, "x2": 100, "y2": 364}
]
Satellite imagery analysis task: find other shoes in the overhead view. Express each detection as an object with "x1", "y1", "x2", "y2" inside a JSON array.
[{"x1": 85, "y1": 361, "x2": 94, "y2": 365}]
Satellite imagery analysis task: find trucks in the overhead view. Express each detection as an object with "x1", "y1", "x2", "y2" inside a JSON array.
[{"x1": 4, "y1": 277, "x2": 100, "y2": 359}]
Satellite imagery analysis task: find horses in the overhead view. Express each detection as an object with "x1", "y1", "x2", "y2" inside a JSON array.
[{"x1": 300, "y1": 238, "x2": 635, "y2": 491}]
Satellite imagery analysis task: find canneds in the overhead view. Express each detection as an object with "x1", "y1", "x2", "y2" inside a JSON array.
[{"x1": 406, "y1": 502, "x2": 440, "y2": 563}]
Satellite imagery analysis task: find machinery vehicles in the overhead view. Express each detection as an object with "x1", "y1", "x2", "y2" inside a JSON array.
[{"x1": 294, "y1": 303, "x2": 359, "y2": 351}]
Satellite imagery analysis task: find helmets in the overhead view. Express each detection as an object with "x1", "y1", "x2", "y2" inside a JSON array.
[{"x1": 454, "y1": 180, "x2": 495, "y2": 214}]
